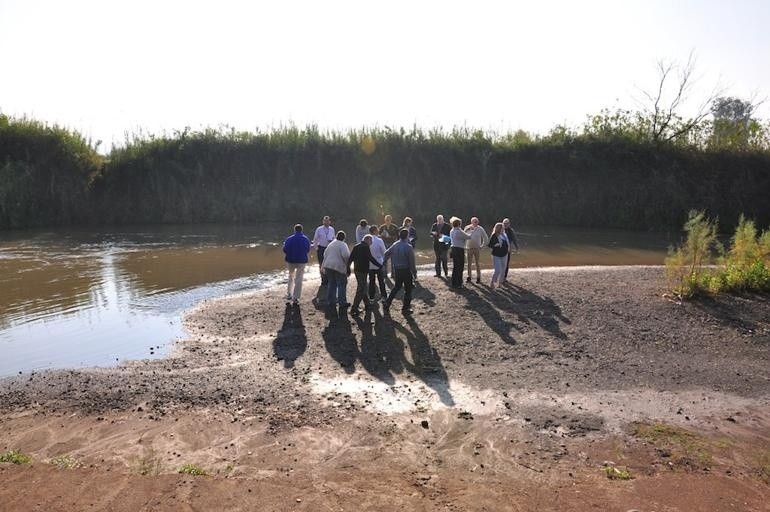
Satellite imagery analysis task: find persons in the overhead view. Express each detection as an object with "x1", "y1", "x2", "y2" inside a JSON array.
[
  {"x1": 313, "y1": 215, "x2": 416, "y2": 315},
  {"x1": 431, "y1": 214, "x2": 520, "y2": 286},
  {"x1": 283, "y1": 224, "x2": 310, "y2": 303}
]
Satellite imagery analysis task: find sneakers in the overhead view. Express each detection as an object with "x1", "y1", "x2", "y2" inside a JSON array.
[
  {"x1": 285, "y1": 274, "x2": 419, "y2": 315},
  {"x1": 434, "y1": 272, "x2": 509, "y2": 292}
]
